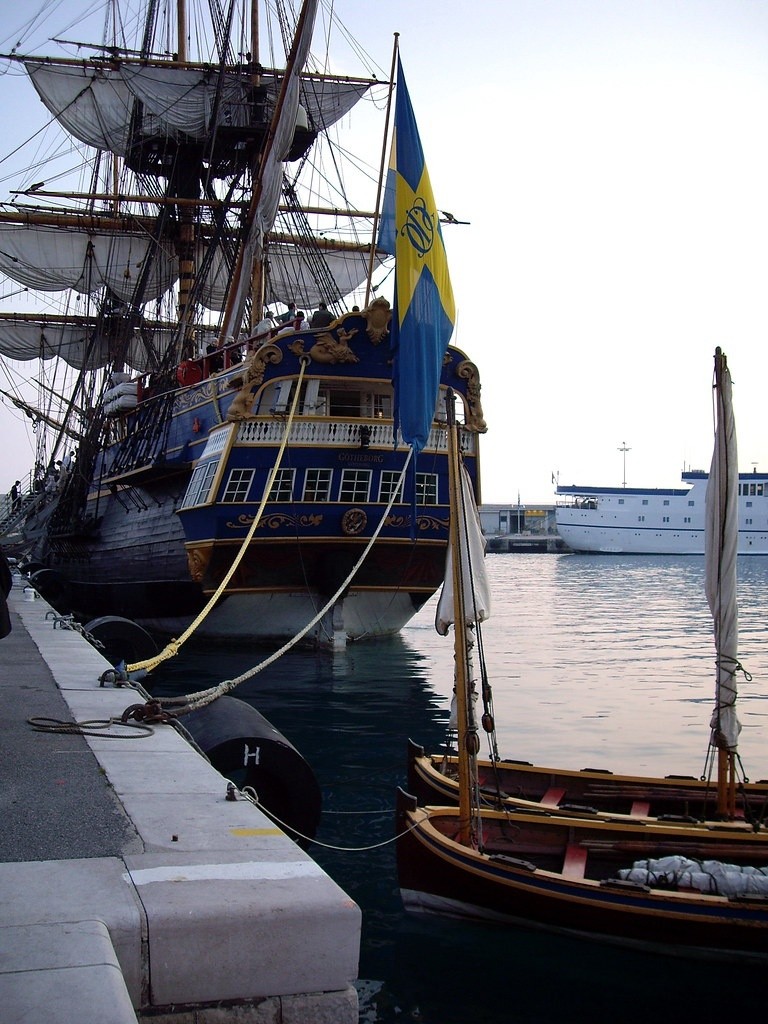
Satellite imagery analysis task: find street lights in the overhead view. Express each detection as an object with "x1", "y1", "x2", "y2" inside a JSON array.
[{"x1": 616, "y1": 441, "x2": 632, "y2": 488}]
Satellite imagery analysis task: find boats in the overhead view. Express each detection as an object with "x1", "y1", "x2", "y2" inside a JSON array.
[{"x1": 552, "y1": 459, "x2": 768, "y2": 556}]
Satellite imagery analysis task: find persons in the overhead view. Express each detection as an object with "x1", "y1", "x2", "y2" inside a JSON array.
[
  {"x1": 0, "y1": 544, "x2": 13, "y2": 639},
  {"x1": 11, "y1": 451, "x2": 74, "y2": 509},
  {"x1": 206, "y1": 300, "x2": 361, "y2": 375}
]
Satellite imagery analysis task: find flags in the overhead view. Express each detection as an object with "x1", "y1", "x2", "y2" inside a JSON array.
[{"x1": 377, "y1": 46, "x2": 454, "y2": 445}]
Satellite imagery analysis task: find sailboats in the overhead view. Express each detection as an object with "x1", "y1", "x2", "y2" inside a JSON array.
[
  {"x1": 398, "y1": 381, "x2": 768, "y2": 951},
  {"x1": 0, "y1": 0, "x2": 491, "y2": 652}
]
